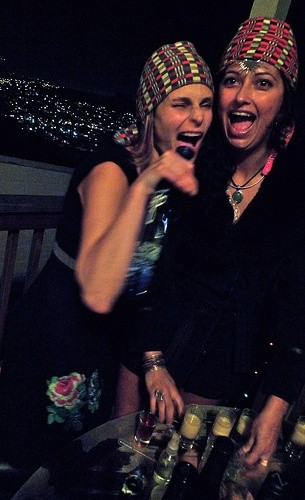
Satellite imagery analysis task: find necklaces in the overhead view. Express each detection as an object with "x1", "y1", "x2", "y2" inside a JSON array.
[
  {"x1": 153, "y1": 143, "x2": 163, "y2": 156},
  {"x1": 226, "y1": 161, "x2": 269, "y2": 206}
]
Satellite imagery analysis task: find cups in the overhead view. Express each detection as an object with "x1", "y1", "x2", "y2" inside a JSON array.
[
  {"x1": 236, "y1": 408, "x2": 256, "y2": 439},
  {"x1": 176, "y1": 404, "x2": 206, "y2": 450},
  {"x1": 208, "y1": 407, "x2": 236, "y2": 441},
  {"x1": 285, "y1": 416, "x2": 305, "y2": 460},
  {"x1": 134, "y1": 409, "x2": 158, "y2": 445}
]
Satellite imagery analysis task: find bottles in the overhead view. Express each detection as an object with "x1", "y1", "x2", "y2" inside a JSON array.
[
  {"x1": 153, "y1": 432, "x2": 182, "y2": 485},
  {"x1": 117, "y1": 436, "x2": 241, "y2": 500},
  {"x1": 253, "y1": 471, "x2": 289, "y2": 500}
]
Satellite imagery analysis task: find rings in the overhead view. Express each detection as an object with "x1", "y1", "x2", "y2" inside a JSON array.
[
  {"x1": 259, "y1": 457, "x2": 270, "y2": 470},
  {"x1": 155, "y1": 390, "x2": 164, "y2": 400}
]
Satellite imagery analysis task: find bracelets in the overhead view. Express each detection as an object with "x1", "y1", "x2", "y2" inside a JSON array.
[{"x1": 142, "y1": 354, "x2": 166, "y2": 371}]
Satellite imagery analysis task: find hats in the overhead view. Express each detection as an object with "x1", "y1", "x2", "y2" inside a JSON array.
[
  {"x1": 216, "y1": 17, "x2": 299, "y2": 91},
  {"x1": 136, "y1": 40, "x2": 214, "y2": 120}
]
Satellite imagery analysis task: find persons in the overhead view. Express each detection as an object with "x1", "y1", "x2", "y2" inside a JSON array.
[
  {"x1": 8, "y1": 40, "x2": 215, "y2": 426},
  {"x1": 194, "y1": 16, "x2": 305, "y2": 481}
]
